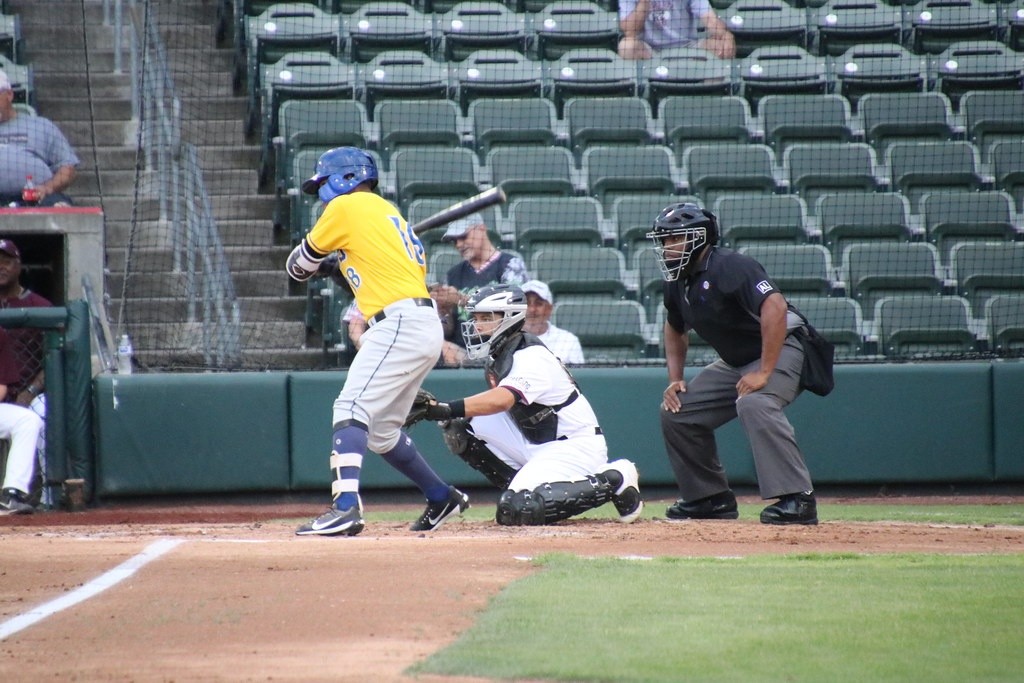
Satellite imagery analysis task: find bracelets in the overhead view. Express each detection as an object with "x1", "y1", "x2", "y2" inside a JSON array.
[{"x1": 27, "y1": 384, "x2": 39, "y2": 397}]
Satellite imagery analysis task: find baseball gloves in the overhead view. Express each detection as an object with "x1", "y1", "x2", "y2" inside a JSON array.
[{"x1": 403, "y1": 385, "x2": 436, "y2": 428}]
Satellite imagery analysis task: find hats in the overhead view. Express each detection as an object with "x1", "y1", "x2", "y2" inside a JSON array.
[
  {"x1": 0, "y1": 70, "x2": 12, "y2": 91},
  {"x1": 0, "y1": 239, "x2": 20, "y2": 259},
  {"x1": 521, "y1": 279, "x2": 552, "y2": 305},
  {"x1": 441, "y1": 213, "x2": 484, "y2": 241}
]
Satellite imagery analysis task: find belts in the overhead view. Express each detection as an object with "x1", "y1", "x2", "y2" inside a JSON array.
[{"x1": 557, "y1": 427, "x2": 603, "y2": 441}]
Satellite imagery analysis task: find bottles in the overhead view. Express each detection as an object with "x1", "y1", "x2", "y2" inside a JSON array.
[
  {"x1": 21, "y1": 176, "x2": 37, "y2": 208},
  {"x1": 118, "y1": 334, "x2": 132, "y2": 375}
]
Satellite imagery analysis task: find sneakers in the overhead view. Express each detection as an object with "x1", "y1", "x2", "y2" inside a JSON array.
[
  {"x1": 409, "y1": 485, "x2": 472, "y2": 531},
  {"x1": 0, "y1": 487, "x2": 35, "y2": 516},
  {"x1": 610, "y1": 459, "x2": 643, "y2": 524},
  {"x1": 295, "y1": 506, "x2": 365, "y2": 537}
]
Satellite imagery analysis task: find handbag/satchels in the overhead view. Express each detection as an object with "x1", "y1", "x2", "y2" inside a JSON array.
[{"x1": 786, "y1": 302, "x2": 835, "y2": 398}]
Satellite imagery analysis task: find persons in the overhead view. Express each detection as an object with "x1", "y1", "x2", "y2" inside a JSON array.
[
  {"x1": 618, "y1": 0, "x2": 736, "y2": 59},
  {"x1": 402, "y1": 284, "x2": 644, "y2": 526},
  {"x1": 286, "y1": 147, "x2": 472, "y2": 536},
  {"x1": 0, "y1": 70, "x2": 81, "y2": 208},
  {"x1": 0, "y1": 240, "x2": 64, "y2": 515},
  {"x1": 343, "y1": 213, "x2": 584, "y2": 369},
  {"x1": 645, "y1": 202, "x2": 819, "y2": 524}
]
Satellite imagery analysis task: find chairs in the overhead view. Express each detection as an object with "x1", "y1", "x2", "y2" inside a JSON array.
[
  {"x1": 0, "y1": 0, "x2": 38, "y2": 117},
  {"x1": 234, "y1": 0, "x2": 1024, "y2": 363}
]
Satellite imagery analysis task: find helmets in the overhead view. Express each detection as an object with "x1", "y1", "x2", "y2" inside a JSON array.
[
  {"x1": 460, "y1": 284, "x2": 528, "y2": 355},
  {"x1": 302, "y1": 146, "x2": 378, "y2": 204},
  {"x1": 646, "y1": 202, "x2": 719, "y2": 283}
]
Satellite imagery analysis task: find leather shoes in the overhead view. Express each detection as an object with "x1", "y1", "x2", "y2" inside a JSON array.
[
  {"x1": 358, "y1": 297, "x2": 438, "y2": 333},
  {"x1": 760, "y1": 493, "x2": 819, "y2": 525},
  {"x1": 665, "y1": 489, "x2": 739, "y2": 520}
]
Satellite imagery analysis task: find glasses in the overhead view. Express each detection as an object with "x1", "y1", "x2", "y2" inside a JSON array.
[{"x1": 452, "y1": 225, "x2": 479, "y2": 241}]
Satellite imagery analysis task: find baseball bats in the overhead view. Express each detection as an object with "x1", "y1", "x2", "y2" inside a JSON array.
[{"x1": 412, "y1": 186, "x2": 507, "y2": 234}]
